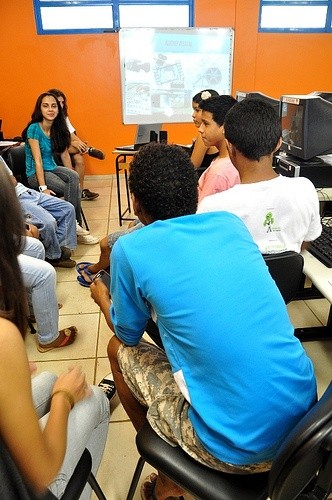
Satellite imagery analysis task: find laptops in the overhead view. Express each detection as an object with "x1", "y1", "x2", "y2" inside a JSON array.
[{"x1": 115, "y1": 124, "x2": 162, "y2": 151}]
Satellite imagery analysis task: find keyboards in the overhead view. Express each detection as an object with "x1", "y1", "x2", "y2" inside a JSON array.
[{"x1": 308, "y1": 222, "x2": 332, "y2": 270}]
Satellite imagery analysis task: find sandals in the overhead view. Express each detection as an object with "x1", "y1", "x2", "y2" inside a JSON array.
[{"x1": 76, "y1": 261, "x2": 93, "y2": 287}]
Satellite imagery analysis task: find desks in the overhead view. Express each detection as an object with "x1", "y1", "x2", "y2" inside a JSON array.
[
  {"x1": 112, "y1": 149, "x2": 143, "y2": 227},
  {"x1": 291, "y1": 187, "x2": 332, "y2": 345}
]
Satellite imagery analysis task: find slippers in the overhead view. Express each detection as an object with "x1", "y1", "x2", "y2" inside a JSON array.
[
  {"x1": 30, "y1": 304, "x2": 63, "y2": 323},
  {"x1": 37, "y1": 327, "x2": 71, "y2": 353}
]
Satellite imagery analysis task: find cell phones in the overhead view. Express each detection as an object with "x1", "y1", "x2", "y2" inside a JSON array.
[{"x1": 93, "y1": 269, "x2": 111, "y2": 299}]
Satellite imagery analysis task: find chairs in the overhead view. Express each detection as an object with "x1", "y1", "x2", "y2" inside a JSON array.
[
  {"x1": 7, "y1": 144, "x2": 90, "y2": 232},
  {"x1": 125, "y1": 379, "x2": 332, "y2": 500},
  {"x1": 0, "y1": 433, "x2": 107, "y2": 500},
  {"x1": 261, "y1": 250, "x2": 306, "y2": 308}
]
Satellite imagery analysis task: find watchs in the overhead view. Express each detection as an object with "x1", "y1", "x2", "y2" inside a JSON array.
[{"x1": 38, "y1": 184, "x2": 48, "y2": 192}]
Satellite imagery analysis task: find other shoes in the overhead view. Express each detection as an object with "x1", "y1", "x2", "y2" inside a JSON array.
[{"x1": 51, "y1": 246, "x2": 76, "y2": 268}]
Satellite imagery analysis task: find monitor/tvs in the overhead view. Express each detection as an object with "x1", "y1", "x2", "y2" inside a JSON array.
[
  {"x1": 279, "y1": 94, "x2": 332, "y2": 161},
  {"x1": 235, "y1": 90, "x2": 280, "y2": 110}
]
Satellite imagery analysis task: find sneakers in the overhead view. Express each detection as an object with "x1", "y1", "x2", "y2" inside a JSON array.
[
  {"x1": 75, "y1": 221, "x2": 99, "y2": 244},
  {"x1": 98, "y1": 371, "x2": 121, "y2": 416},
  {"x1": 82, "y1": 188, "x2": 99, "y2": 201},
  {"x1": 88, "y1": 146, "x2": 105, "y2": 160}
]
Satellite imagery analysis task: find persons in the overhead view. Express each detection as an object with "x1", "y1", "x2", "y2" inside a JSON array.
[
  {"x1": 11, "y1": 232, "x2": 78, "y2": 354},
  {"x1": 0, "y1": 153, "x2": 77, "y2": 269},
  {"x1": 196, "y1": 98, "x2": 324, "y2": 254},
  {"x1": 74, "y1": 95, "x2": 242, "y2": 288},
  {"x1": 48, "y1": 88, "x2": 106, "y2": 202},
  {"x1": 20, "y1": 219, "x2": 64, "y2": 310},
  {"x1": 22, "y1": 92, "x2": 101, "y2": 245},
  {"x1": 185, "y1": 88, "x2": 222, "y2": 180},
  {"x1": 0, "y1": 156, "x2": 110, "y2": 500},
  {"x1": 89, "y1": 138, "x2": 319, "y2": 500}
]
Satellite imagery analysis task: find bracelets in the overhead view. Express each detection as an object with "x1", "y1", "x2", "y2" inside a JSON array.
[{"x1": 51, "y1": 389, "x2": 75, "y2": 410}]
